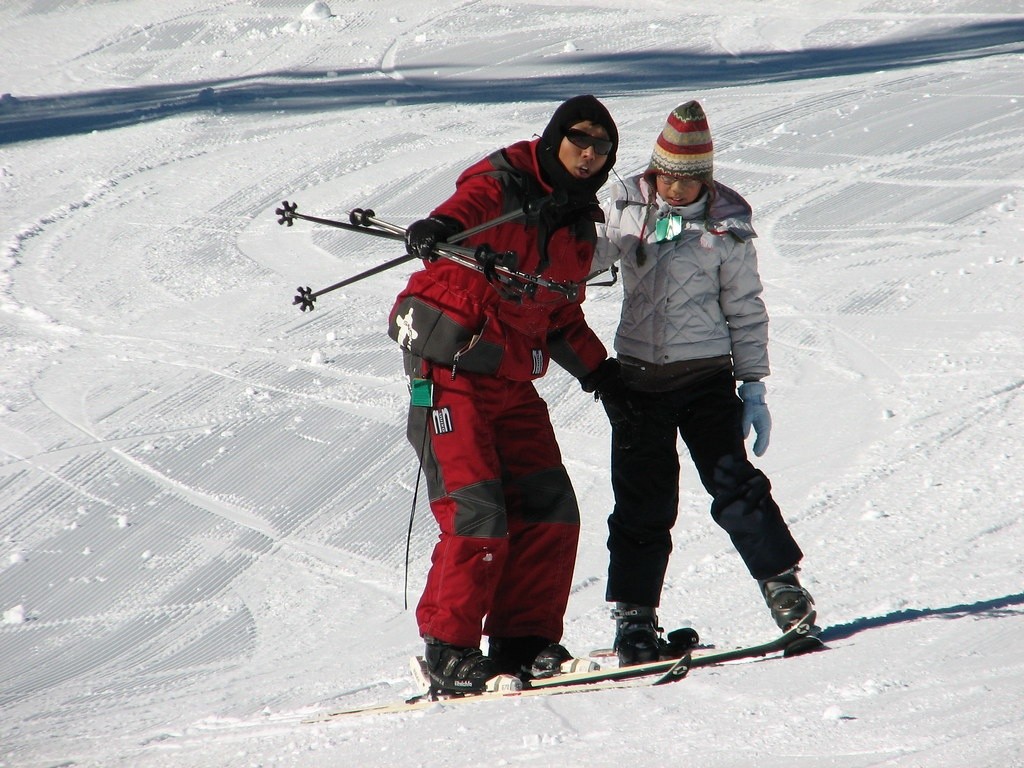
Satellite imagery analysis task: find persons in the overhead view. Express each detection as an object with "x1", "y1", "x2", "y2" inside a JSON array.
[
  {"x1": 589, "y1": 100, "x2": 815, "y2": 667},
  {"x1": 388, "y1": 95, "x2": 643, "y2": 696}
]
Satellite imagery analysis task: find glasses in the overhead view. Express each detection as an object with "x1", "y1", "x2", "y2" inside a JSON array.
[
  {"x1": 657, "y1": 173, "x2": 702, "y2": 187},
  {"x1": 565, "y1": 129, "x2": 613, "y2": 155}
]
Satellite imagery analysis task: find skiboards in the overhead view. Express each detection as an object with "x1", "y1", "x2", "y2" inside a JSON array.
[{"x1": 301, "y1": 606, "x2": 818, "y2": 727}]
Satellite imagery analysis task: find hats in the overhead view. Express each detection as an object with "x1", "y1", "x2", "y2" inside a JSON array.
[{"x1": 636, "y1": 100, "x2": 729, "y2": 265}]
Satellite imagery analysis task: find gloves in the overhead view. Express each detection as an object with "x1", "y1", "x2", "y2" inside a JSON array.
[
  {"x1": 405, "y1": 215, "x2": 458, "y2": 264},
  {"x1": 739, "y1": 382, "x2": 771, "y2": 457},
  {"x1": 591, "y1": 357, "x2": 642, "y2": 450}
]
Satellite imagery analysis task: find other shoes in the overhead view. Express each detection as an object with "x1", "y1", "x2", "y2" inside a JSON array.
[
  {"x1": 613, "y1": 603, "x2": 660, "y2": 664},
  {"x1": 488, "y1": 636, "x2": 573, "y2": 677},
  {"x1": 759, "y1": 572, "x2": 812, "y2": 630},
  {"x1": 424, "y1": 638, "x2": 498, "y2": 692}
]
